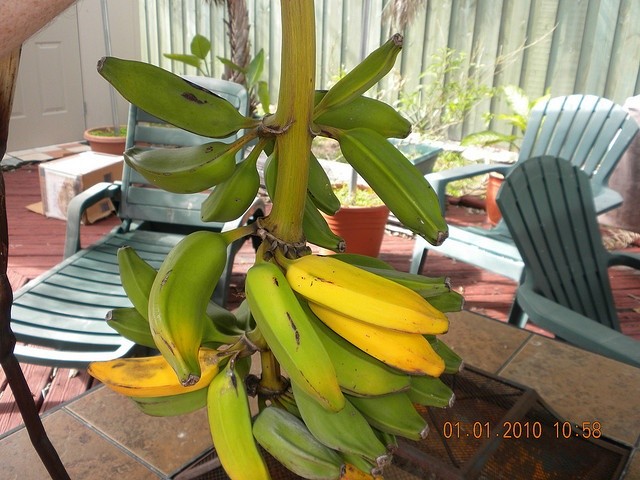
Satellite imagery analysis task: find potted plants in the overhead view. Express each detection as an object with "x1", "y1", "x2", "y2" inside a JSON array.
[
  {"x1": 320, "y1": 67, "x2": 390, "y2": 257},
  {"x1": 459, "y1": 85, "x2": 553, "y2": 226},
  {"x1": 390, "y1": 26, "x2": 558, "y2": 178}
]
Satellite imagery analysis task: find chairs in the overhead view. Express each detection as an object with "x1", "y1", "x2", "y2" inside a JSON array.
[
  {"x1": 410, "y1": 94, "x2": 639, "y2": 328},
  {"x1": 11, "y1": 75, "x2": 249, "y2": 368},
  {"x1": 495, "y1": 154, "x2": 640, "y2": 369}
]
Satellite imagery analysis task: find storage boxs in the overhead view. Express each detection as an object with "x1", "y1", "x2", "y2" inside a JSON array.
[{"x1": 26, "y1": 151, "x2": 124, "y2": 225}]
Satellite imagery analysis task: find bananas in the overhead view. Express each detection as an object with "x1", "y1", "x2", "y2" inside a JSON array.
[{"x1": 87, "y1": 34, "x2": 465, "y2": 480}]
